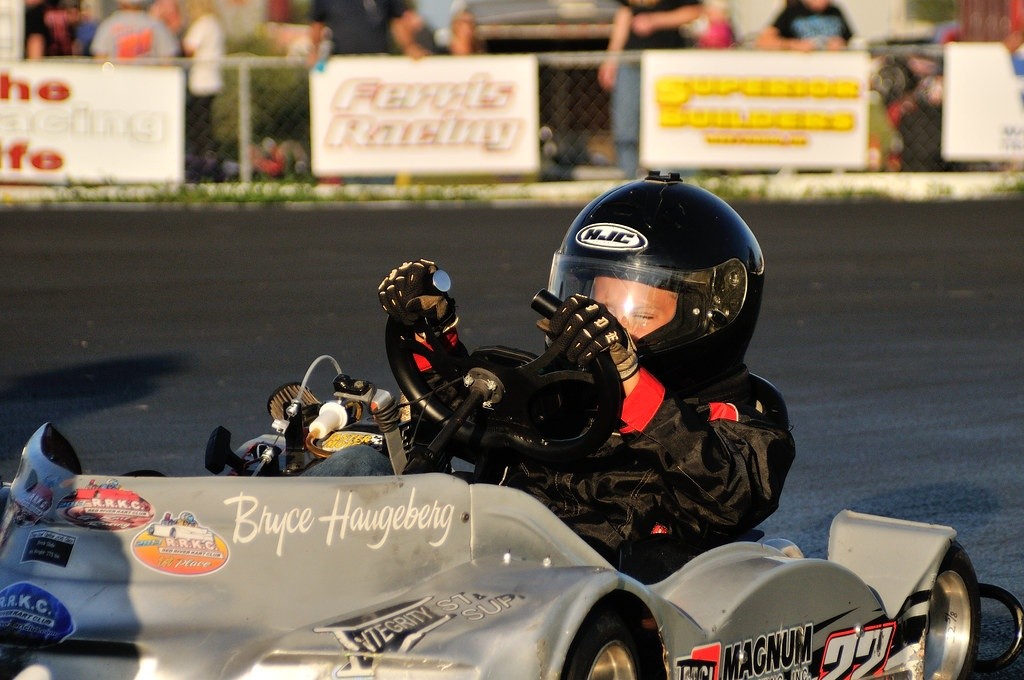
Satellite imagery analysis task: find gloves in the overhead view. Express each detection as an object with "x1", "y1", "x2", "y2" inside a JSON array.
[
  {"x1": 375, "y1": 256, "x2": 461, "y2": 335},
  {"x1": 538, "y1": 292, "x2": 640, "y2": 381}
]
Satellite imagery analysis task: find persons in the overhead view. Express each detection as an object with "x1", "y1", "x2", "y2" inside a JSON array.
[
  {"x1": 759, "y1": 0, "x2": 853, "y2": 49},
  {"x1": 25, "y1": 0, "x2": 222, "y2": 183},
  {"x1": 309, "y1": 0, "x2": 435, "y2": 70},
  {"x1": 299, "y1": 170, "x2": 796, "y2": 585},
  {"x1": 598, "y1": 0, "x2": 705, "y2": 179}
]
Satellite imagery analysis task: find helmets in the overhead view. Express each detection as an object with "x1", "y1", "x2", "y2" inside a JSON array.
[{"x1": 548, "y1": 169, "x2": 765, "y2": 386}]
